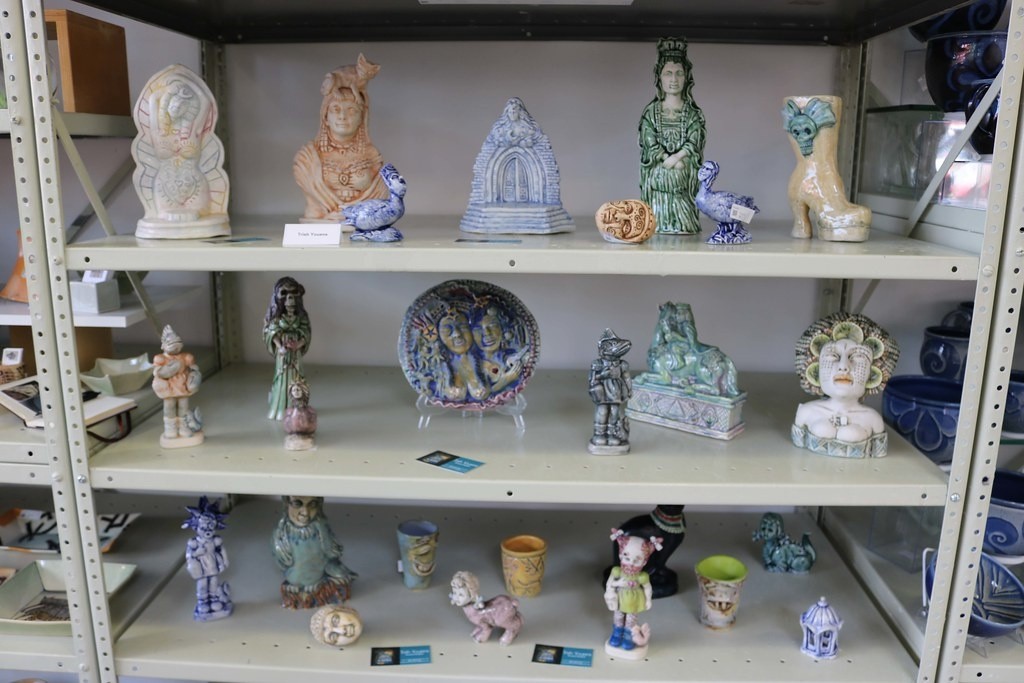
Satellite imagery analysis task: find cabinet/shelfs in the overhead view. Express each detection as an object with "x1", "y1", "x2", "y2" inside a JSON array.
[{"x1": 0, "y1": 1, "x2": 1024, "y2": 683}]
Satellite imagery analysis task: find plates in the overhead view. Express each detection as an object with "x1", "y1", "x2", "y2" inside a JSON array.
[{"x1": 0, "y1": 507, "x2": 141, "y2": 555}]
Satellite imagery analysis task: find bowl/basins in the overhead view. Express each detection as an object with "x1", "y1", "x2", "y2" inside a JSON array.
[
  {"x1": 881, "y1": 325, "x2": 1024, "y2": 637},
  {"x1": 0, "y1": 559, "x2": 138, "y2": 636},
  {"x1": 79, "y1": 352, "x2": 153, "y2": 396},
  {"x1": 924, "y1": 31, "x2": 1007, "y2": 105}
]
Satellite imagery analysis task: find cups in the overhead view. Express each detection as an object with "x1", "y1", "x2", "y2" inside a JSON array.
[
  {"x1": 500, "y1": 534, "x2": 547, "y2": 598},
  {"x1": 694, "y1": 554, "x2": 747, "y2": 630},
  {"x1": 397, "y1": 519, "x2": 440, "y2": 590}
]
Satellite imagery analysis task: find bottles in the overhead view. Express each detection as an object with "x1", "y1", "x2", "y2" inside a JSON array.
[{"x1": 966, "y1": 79, "x2": 999, "y2": 156}]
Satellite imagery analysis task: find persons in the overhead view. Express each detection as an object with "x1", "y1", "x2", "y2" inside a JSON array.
[
  {"x1": 604, "y1": 528, "x2": 663, "y2": 649},
  {"x1": 310, "y1": 603, "x2": 363, "y2": 647},
  {"x1": 595, "y1": 199, "x2": 656, "y2": 243},
  {"x1": 450, "y1": 571, "x2": 484, "y2": 609},
  {"x1": 288, "y1": 379, "x2": 310, "y2": 405},
  {"x1": 758, "y1": 513, "x2": 783, "y2": 540},
  {"x1": 262, "y1": 277, "x2": 312, "y2": 420},
  {"x1": 147, "y1": 76, "x2": 208, "y2": 210},
  {"x1": 294, "y1": 87, "x2": 389, "y2": 221},
  {"x1": 178, "y1": 495, "x2": 229, "y2": 613},
  {"x1": 636, "y1": 37, "x2": 707, "y2": 232},
  {"x1": 796, "y1": 311, "x2": 901, "y2": 444},
  {"x1": 283, "y1": 495, "x2": 323, "y2": 527}
]
